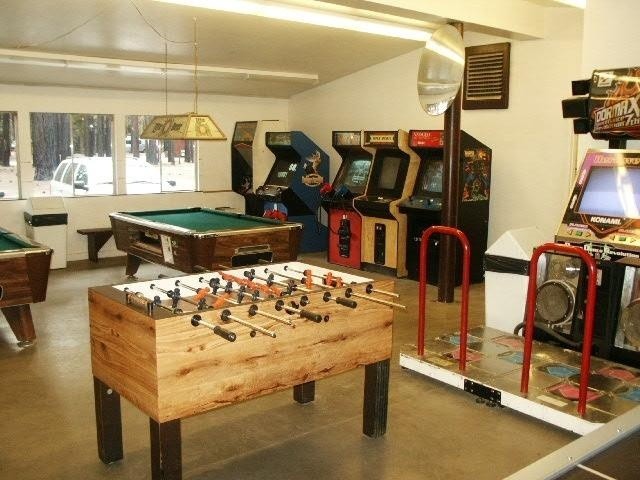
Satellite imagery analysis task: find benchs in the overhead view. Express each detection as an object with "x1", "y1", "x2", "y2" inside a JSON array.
[{"x1": 77, "y1": 227, "x2": 116, "y2": 266}]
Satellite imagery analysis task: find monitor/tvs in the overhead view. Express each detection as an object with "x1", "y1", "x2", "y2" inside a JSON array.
[
  {"x1": 268, "y1": 158, "x2": 298, "y2": 186},
  {"x1": 344, "y1": 159, "x2": 372, "y2": 193},
  {"x1": 578, "y1": 166, "x2": 640, "y2": 219},
  {"x1": 236, "y1": 149, "x2": 252, "y2": 177},
  {"x1": 421, "y1": 159, "x2": 443, "y2": 193},
  {"x1": 376, "y1": 156, "x2": 401, "y2": 190}
]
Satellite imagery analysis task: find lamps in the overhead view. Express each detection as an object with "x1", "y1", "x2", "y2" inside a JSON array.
[{"x1": 137, "y1": 17, "x2": 229, "y2": 142}]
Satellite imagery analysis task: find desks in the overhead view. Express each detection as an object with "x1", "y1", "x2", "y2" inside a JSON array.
[
  {"x1": 109, "y1": 205, "x2": 305, "y2": 279},
  {"x1": 0, "y1": 226, "x2": 55, "y2": 344}
]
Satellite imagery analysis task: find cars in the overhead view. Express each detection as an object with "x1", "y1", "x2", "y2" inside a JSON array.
[
  {"x1": 51, "y1": 156, "x2": 177, "y2": 195},
  {"x1": 125, "y1": 137, "x2": 147, "y2": 153}
]
